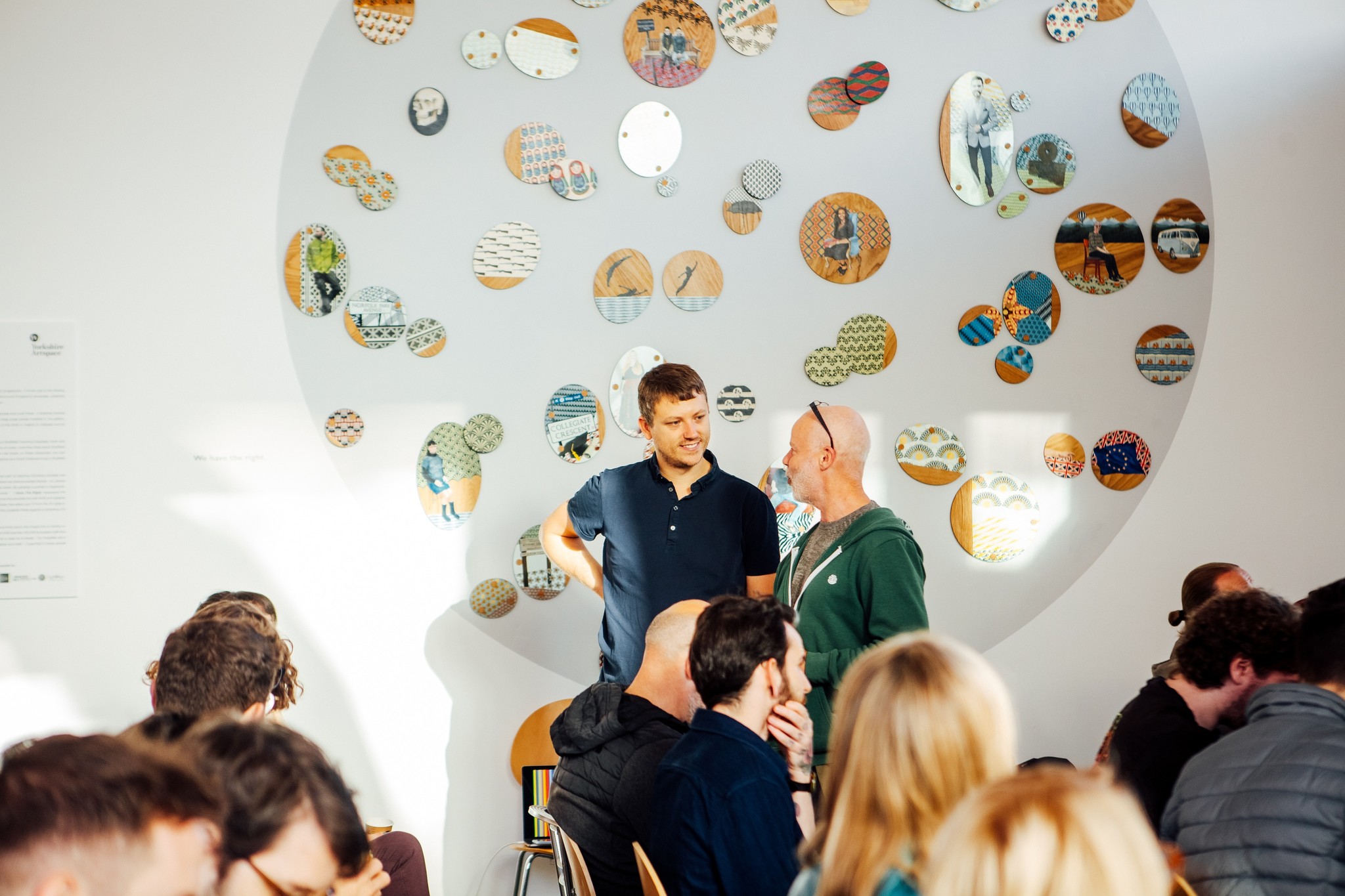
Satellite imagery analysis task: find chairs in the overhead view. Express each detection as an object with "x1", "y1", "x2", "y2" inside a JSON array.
[{"x1": 510, "y1": 698, "x2": 666, "y2": 896}]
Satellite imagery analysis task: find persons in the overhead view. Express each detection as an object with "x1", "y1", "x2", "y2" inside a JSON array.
[
  {"x1": 641, "y1": 593, "x2": 818, "y2": 896},
  {"x1": 660, "y1": 26, "x2": 687, "y2": 74},
  {"x1": 420, "y1": 441, "x2": 460, "y2": 521},
  {"x1": 306, "y1": 226, "x2": 340, "y2": 314},
  {"x1": 1, "y1": 589, "x2": 430, "y2": 896},
  {"x1": 545, "y1": 599, "x2": 711, "y2": 896},
  {"x1": 537, "y1": 364, "x2": 779, "y2": 709},
  {"x1": 963, "y1": 75, "x2": 998, "y2": 197},
  {"x1": 786, "y1": 562, "x2": 1345, "y2": 896},
  {"x1": 767, "y1": 405, "x2": 930, "y2": 779},
  {"x1": 823, "y1": 207, "x2": 854, "y2": 277},
  {"x1": 1084, "y1": 218, "x2": 1127, "y2": 283}
]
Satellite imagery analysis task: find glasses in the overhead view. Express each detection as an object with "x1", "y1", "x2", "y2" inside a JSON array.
[{"x1": 807, "y1": 400, "x2": 834, "y2": 450}]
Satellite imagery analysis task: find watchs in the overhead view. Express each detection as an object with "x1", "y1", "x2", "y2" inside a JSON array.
[{"x1": 788, "y1": 770, "x2": 820, "y2": 797}]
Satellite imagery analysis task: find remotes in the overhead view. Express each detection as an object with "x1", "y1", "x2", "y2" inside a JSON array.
[{"x1": 532, "y1": 839, "x2": 552, "y2": 844}]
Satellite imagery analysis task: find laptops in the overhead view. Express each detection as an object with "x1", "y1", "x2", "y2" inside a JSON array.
[{"x1": 522, "y1": 765, "x2": 557, "y2": 848}]
